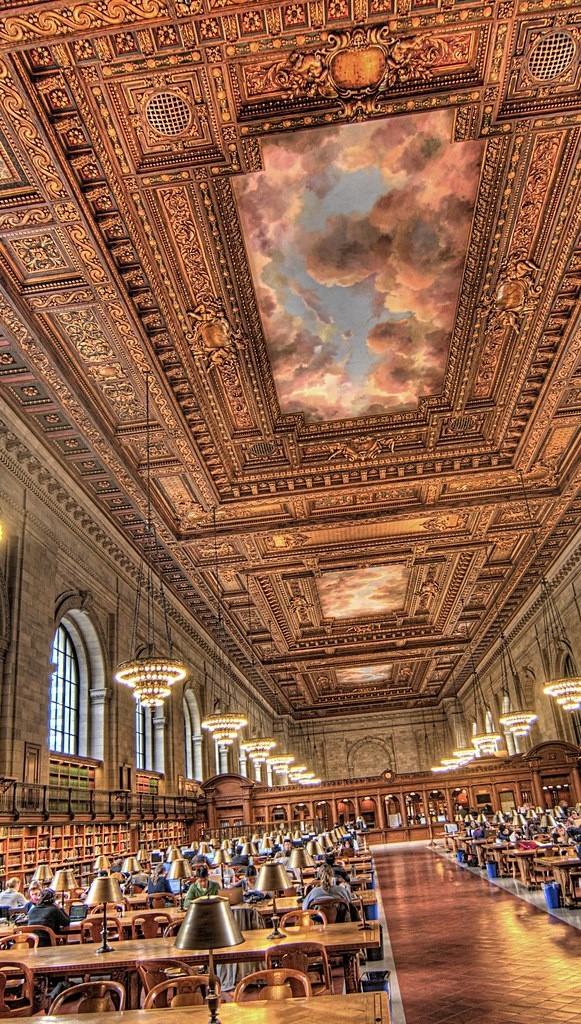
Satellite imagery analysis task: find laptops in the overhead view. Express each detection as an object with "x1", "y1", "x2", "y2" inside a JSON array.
[
  {"x1": 69, "y1": 904, "x2": 88, "y2": 922},
  {"x1": 484, "y1": 830, "x2": 496, "y2": 839},
  {"x1": 208, "y1": 874, "x2": 223, "y2": 886},
  {"x1": 166, "y1": 878, "x2": 181, "y2": 895}
]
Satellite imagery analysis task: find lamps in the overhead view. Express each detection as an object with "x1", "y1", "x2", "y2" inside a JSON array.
[
  {"x1": 435, "y1": 661, "x2": 461, "y2": 769},
  {"x1": 200, "y1": 507, "x2": 249, "y2": 747},
  {"x1": 241, "y1": 574, "x2": 276, "y2": 763},
  {"x1": 266, "y1": 621, "x2": 320, "y2": 785},
  {"x1": 455, "y1": 803, "x2": 581, "y2": 844},
  {"x1": 113, "y1": 369, "x2": 188, "y2": 709},
  {"x1": 418, "y1": 694, "x2": 449, "y2": 772},
  {"x1": 461, "y1": 599, "x2": 501, "y2": 753},
  {"x1": 517, "y1": 470, "x2": 581, "y2": 710},
  {"x1": 485, "y1": 548, "x2": 536, "y2": 737},
  {"x1": 446, "y1": 634, "x2": 475, "y2": 760},
  {"x1": 0, "y1": 825, "x2": 355, "y2": 1024}
]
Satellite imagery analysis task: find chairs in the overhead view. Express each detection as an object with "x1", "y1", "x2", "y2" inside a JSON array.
[{"x1": 486, "y1": 835, "x2": 581, "y2": 906}]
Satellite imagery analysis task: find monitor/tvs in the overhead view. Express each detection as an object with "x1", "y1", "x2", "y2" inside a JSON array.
[{"x1": 150, "y1": 852, "x2": 164, "y2": 867}]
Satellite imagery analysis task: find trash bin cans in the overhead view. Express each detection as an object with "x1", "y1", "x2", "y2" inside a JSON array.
[
  {"x1": 359, "y1": 970, "x2": 392, "y2": 1012},
  {"x1": 364, "y1": 856, "x2": 376, "y2": 889},
  {"x1": 366, "y1": 924, "x2": 384, "y2": 961},
  {"x1": 540, "y1": 881, "x2": 561, "y2": 909},
  {"x1": 365, "y1": 904, "x2": 379, "y2": 921},
  {"x1": 455, "y1": 848, "x2": 498, "y2": 880}
]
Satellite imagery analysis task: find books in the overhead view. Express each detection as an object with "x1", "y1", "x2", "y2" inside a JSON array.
[{"x1": 0, "y1": 819, "x2": 317, "y2": 876}]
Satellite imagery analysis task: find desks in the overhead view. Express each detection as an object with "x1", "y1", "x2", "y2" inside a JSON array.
[
  {"x1": 0, "y1": 920, "x2": 381, "y2": 1015},
  {"x1": 435, "y1": 831, "x2": 460, "y2": 851},
  {"x1": 0, "y1": 890, "x2": 377, "y2": 982},
  {"x1": 445, "y1": 834, "x2": 463, "y2": 853},
  {"x1": 355, "y1": 849, "x2": 372, "y2": 860},
  {"x1": 0, "y1": 991, "x2": 391, "y2": 1024},
  {"x1": 467, "y1": 839, "x2": 487, "y2": 868},
  {"x1": 482, "y1": 839, "x2": 532, "y2": 876},
  {"x1": 533, "y1": 856, "x2": 581, "y2": 910},
  {"x1": 304, "y1": 862, "x2": 372, "y2": 879},
  {"x1": 456, "y1": 837, "x2": 471, "y2": 854},
  {"x1": 319, "y1": 856, "x2": 373, "y2": 874},
  {"x1": 501, "y1": 847, "x2": 568, "y2": 891},
  {"x1": 57, "y1": 873, "x2": 374, "y2": 915}
]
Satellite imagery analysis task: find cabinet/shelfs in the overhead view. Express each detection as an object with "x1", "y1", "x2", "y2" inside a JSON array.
[
  {"x1": 138, "y1": 821, "x2": 189, "y2": 855},
  {"x1": 0, "y1": 825, "x2": 132, "y2": 898}
]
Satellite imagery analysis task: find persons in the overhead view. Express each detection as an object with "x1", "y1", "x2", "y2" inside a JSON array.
[
  {"x1": 232, "y1": 813, "x2": 357, "y2": 922},
  {"x1": 0, "y1": 844, "x2": 249, "y2": 953},
  {"x1": 456, "y1": 800, "x2": 581, "y2": 863}
]
